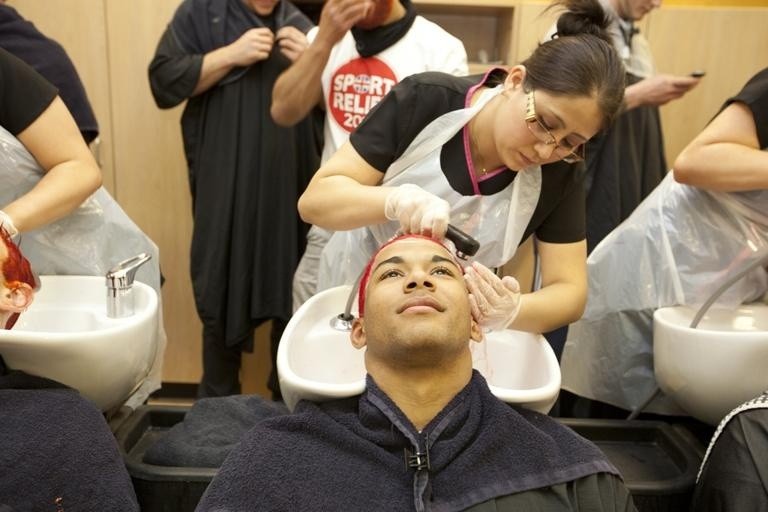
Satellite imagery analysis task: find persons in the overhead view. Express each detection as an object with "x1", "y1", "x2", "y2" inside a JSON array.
[
  {"x1": 270, "y1": 0, "x2": 470, "y2": 317},
  {"x1": 0, "y1": 47, "x2": 168, "y2": 412},
  {"x1": 193, "y1": 234, "x2": 638, "y2": 511},
  {"x1": 147, "y1": 0, "x2": 324, "y2": 399},
  {"x1": 0, "y1": 4, "x2": 100, "y2": 165},
  {"x1": 694, "y1": 392, "x2": 768, "y2": 511},
  {"x1": 540, "y1": 0, "x2": 706, "y2": 364},
  {"x1": 1, "y1": 223, "x2": 144, "y2": 512},
  {"x1": 559, "y1": 66, "x2": 768, "y2": 419},
  {"x1": 298, "y1": 0, "x2": 628, "y2": 335}
]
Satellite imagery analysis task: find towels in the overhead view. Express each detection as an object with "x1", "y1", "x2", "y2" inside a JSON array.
[
  {"x1": 143, "y1": 394, "x2": 290, "y2": 468},
  {"x1": 193, "y1": 368, "x2": 624, "y2": 512},
  {"x1": 0, "y1": 389, "x2": 140, "y2": 512}
]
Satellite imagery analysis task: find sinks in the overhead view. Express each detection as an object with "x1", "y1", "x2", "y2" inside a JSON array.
[
  {"x1": 276, "y1": 284, "x2": 562, "y2": 415},
  {"x1": 1, "y1": 276, "x2": 158, "y2": 412},
  {"x1": 654, "y1": 304, "x2": 768, "y2": 428}
]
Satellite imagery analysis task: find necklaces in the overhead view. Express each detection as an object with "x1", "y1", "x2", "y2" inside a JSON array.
[{"x1": 472, "y1": 119, "x2": 499, "y2": 177}]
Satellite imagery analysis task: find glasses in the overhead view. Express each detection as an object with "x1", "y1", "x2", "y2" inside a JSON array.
[{"x1": 524, "y1": 88, "x2": 586, "y2": 164}]
